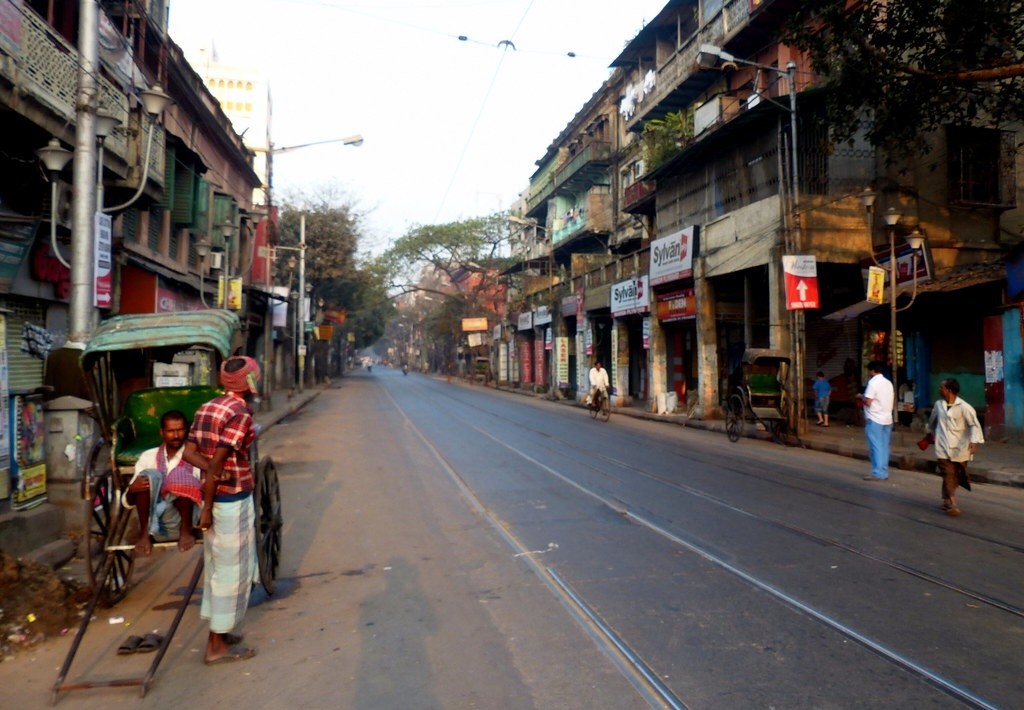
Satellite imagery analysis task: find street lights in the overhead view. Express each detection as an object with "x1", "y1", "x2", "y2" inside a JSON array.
[
  {"x1": 508, "y1": 216, "x2": 558, "y2": 401},
  {"x1": 468, "y1": 261, "x2": 499, "y2": 389},
  {"x1": 290, "y1": 290, "x2": 300, "y2": 390},
  {"x1": 287, "y1": 256, "x2": 299, "y2": 295},
  {"x1": 857, "y1": 186, "x2": 926, "y2": 431},
  {"x1": 36, "y1": 84, "x2": 177, "y2": 334},
  {"x1": 260, "y1": 135, "x2": 364, "y2": 413},
  {"x1": 694, "y1": 44, "x2": 806, "y2": 435}
]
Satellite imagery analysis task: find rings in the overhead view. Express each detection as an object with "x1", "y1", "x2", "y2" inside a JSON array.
[{"x1": 201, "y1": 527, "x2": 208, "y2": 531}]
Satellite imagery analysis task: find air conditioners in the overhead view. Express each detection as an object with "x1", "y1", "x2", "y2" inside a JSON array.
[
  {"x1": 251, "y1": 187, "x2": 268, "y2": 206},
  {"x1": 210, "y1": 252, "x2": 222, "y2": 268},
  {"x1": 635, "y1": 160, "x2": 648, "y2": 179}
]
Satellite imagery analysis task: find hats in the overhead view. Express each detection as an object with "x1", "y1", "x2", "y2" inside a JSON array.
[{"x1": 220, "y1": 355, "x2": 262, "y2": 403}]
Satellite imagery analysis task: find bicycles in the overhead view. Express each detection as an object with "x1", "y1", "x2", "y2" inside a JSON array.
[{"x1": 589, "y1": 386, "x2": 610, "y2": 423}]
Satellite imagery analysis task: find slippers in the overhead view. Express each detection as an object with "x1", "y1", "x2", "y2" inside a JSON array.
[
  {"x1": 205, "y1": 647, "x2": 257, "y2": 665},
  {"x1": 117, "y1": 635, "x2": 144, "y2": 654},
  {"x1": 223, "y1": 634, "x2": 244, "y2": 644},
  {"x1": 137, "y1": 634, "x2": 165, "y2": 653}
]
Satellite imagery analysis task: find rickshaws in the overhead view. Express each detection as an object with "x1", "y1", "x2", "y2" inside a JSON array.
[
  {"x1": 725, "y1": 348, "x2": 806, "y2": 450},
  {"x1": 50, "y1": 309, "x2": 284, "y2": 705}
]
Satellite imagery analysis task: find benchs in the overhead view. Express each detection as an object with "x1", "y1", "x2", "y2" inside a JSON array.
[
  {"x1": 747, "y1": 363, "x2": 780, "y2": 408},
  {"x1": 111, "y1": 385, "x2": 227, "y2": 477}
]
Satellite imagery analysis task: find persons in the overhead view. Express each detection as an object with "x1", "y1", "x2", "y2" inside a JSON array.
[
  {"x1": 120, "y1": 409, "x2": 206, "y2": 556},
  {"x1": 182, "y1": 354, "x2": 263, "y2": 666},
  {"x1": 812, "y1": 371, "x2": 832, "y2": 427},
  {"x1": 587, "y1": 361, "x2": 610, "y2": 416},
  {"x1": 854, "y1": 359, "x2": 894, "y2": 480},
  {"x1": 923, "y1": 378, "x2": 985, "y2": 516}
]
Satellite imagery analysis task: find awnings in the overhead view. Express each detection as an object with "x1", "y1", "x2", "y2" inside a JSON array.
[
  {"x1": 823, "y1": 267, "x2": 1007, "y2": 321},
  {"x1": 640, "y1": 82, "x2": 828, "y2": 182},
  {"x1": 125, "y1": 253, "x2": 297, "y2": 310},
  {"x1": 497, "y1": 254, "x2": 551, "y2": 277}
]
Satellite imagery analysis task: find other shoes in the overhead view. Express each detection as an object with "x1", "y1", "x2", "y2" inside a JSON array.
[
  {"x1": 941, "y1": 504, "x2": 951, "y2": 510},
  {"x1": 864, "y1": 476, "x2": 879, "y2": 480},
  {"x1": 947, "y1": 504, "x2": 960, "y2": 515}
]
